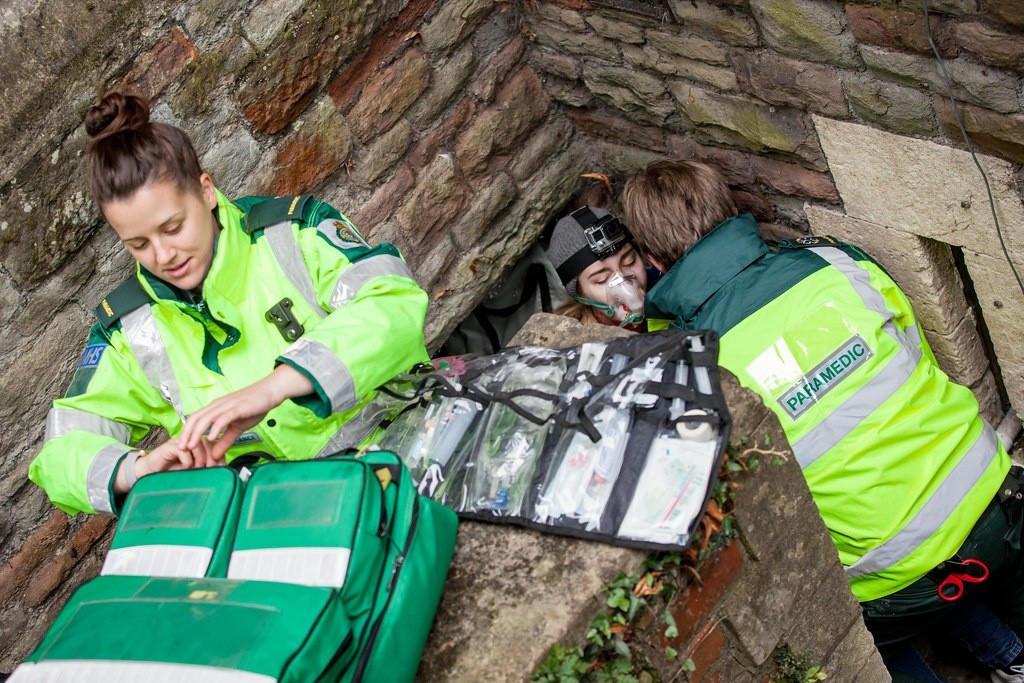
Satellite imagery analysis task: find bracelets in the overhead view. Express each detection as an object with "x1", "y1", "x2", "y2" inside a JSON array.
[{"x1": 122, "y1": 449, "x2": 145, "y2": 490}]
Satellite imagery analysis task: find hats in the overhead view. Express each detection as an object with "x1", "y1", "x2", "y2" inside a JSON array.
[{"x1": 547, "y1": 206, "x2": 642, "y2": 297}]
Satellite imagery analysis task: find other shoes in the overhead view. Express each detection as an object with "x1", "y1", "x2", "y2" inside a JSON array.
[{"x1": 990, "y1": 659, "x2": 1024, "y2": 683}]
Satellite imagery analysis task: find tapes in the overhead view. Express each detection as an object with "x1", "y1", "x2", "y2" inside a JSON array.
[{"x1": 676, "y1": 408, "x2": 712, "y2": 442}]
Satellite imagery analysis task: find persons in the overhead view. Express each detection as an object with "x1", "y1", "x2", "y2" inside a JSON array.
[
  {"x1": 29, "y1": 92, "x2": 432, "y2": 520},
  {"x1": 547, "y1": 159, "x2": 1024, "y2": 683}
]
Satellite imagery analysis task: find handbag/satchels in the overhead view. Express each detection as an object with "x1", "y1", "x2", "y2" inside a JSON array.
[{"x1": 442, "y1": 242, "x2": 576, "y2": 356}]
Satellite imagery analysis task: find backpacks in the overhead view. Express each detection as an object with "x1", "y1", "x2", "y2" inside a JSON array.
[{"x1": 5, "y1": 447, "x2": 458, "y2": 683}]
